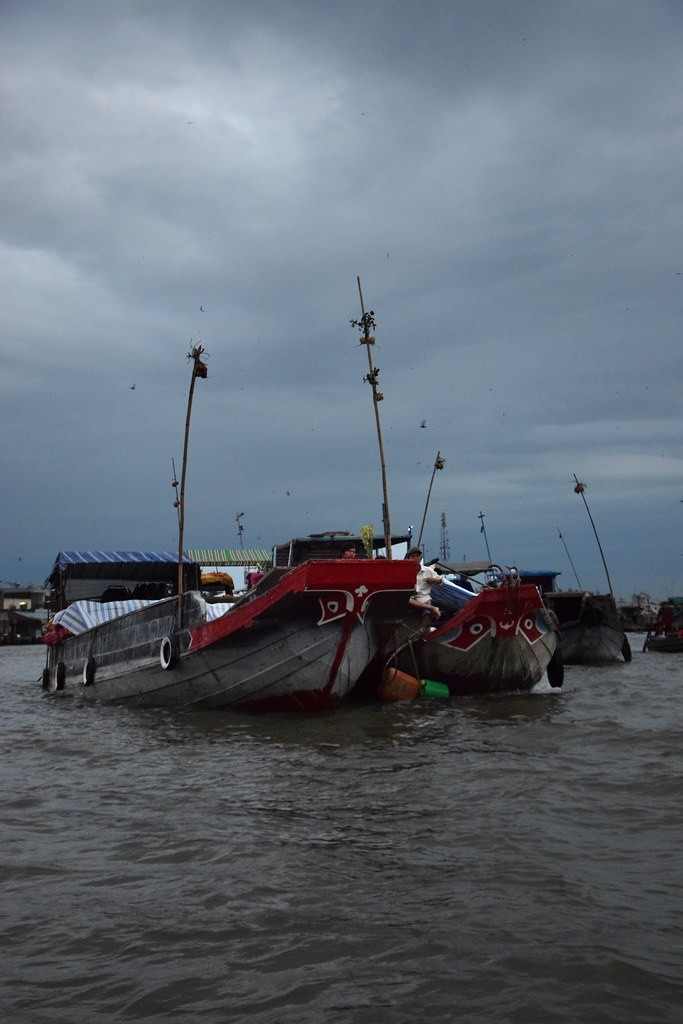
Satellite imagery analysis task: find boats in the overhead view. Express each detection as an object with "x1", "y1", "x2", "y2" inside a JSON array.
[
  {"x1": 345, "y1": 274, "x2": 566, "y2": 701},
  {"x1": 643, "y1": 596, "x2": 683, "y2": 654},
  {"x1": 493, "y1": 564, "x2": 626, "y2": 667},
  {"x1": 37, "y1": 334, "x2": 420, "y2": 711}
]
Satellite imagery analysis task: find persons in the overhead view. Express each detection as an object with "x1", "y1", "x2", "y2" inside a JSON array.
[
  {"x1": 404, "y1": 547, "x2": 444, "y2": 634},
  {"x1": 340, "y1": 543, "x2": 356, "y2": 559},
  {"x1": 654, "y1": 600, "x2": 681, "y2": 638}
]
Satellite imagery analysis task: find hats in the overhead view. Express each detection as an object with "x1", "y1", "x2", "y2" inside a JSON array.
[{"x1": 407, "y1": 547, "x2": 422, "y2": 558}]
